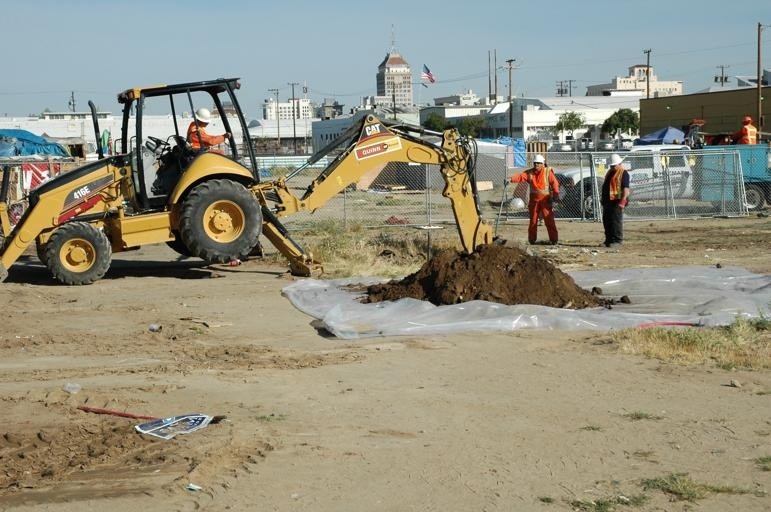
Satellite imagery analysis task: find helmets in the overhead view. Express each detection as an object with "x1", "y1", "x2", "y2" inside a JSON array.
[
  {"x1": 533, "y1": 154, "x2": 545, "y2": 164},
  {"x1": 741, "y1": 116, "x2": 752, "y2": 121},
  {"x1": 608, "y1": 154, "x2": 625, "y2": 164},
  {"x1": 194, "y1": 108, "x2": 210, "y2": 123}
]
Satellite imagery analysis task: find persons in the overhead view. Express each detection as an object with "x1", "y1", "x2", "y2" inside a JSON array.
[
  {"x1": 730, "y1": 117, "x2": 759, "y2": 144},
  {"x1": 600, "y1": 153, "x2": 630, "y2": 247},
  {"x1": 503, "y1": 155, "x2": 560, "y2": 245},
  {"x1": 185, "y1": 108, "x2": 231, "y2": 156}
]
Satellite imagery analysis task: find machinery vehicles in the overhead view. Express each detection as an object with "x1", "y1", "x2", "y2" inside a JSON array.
[{"x1": 0, "y1": 77, "x2": 492, "y2": 286}]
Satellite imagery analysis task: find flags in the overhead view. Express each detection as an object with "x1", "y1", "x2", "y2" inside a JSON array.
[{"x1": 421, "y1": 64, "x2": 437, "y2": 88}]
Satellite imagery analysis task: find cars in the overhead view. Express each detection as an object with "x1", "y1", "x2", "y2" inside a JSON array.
[{"x1": 554, "y1": 137, "x2": 633, "y2": 151}]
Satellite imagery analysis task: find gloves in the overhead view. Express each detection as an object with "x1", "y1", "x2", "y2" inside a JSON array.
[{"x1": 618, "y1": 199, "x2": 627, "y2": 208}]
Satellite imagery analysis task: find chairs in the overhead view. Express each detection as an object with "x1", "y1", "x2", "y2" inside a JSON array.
[{"x1": 173, "y1": 135, "x2": 193, "y2": 153}]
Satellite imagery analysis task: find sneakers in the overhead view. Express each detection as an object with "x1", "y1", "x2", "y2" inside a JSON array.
[
  {"x1": 599, "y1": 241, "x2": 609, "y2": 246},
  {"x1": 530, "y1": 241, "x2": 535, "y2": 244},
  {"x1": 609, "y1": 242, "x2": 623, "y2": 246},
  {"x1": 552, "y1": 241, "x2": 557, "y2": 245}
]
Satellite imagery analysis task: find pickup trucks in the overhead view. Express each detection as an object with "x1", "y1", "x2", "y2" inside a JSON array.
[{"x1": 555, "y1": 143, "x2": 771, "y2": 211}]
